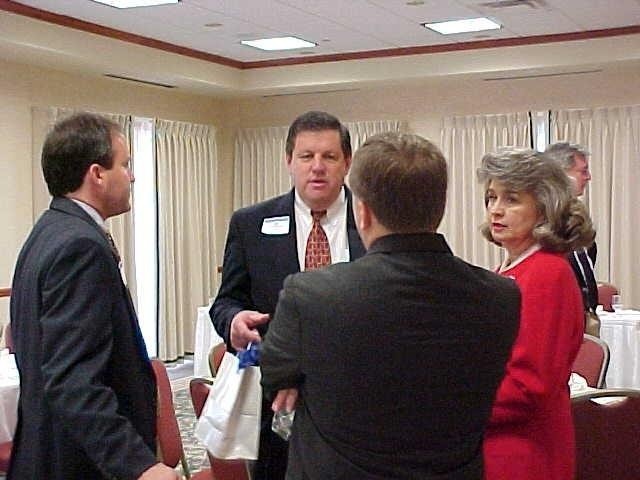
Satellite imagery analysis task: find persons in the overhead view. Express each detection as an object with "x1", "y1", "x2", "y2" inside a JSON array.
[
  {"x1": 6, "y1": 113, "x2": 184, "y2": 480},
  {"x1": 261, "y1": 131, "x2": 521, "y2": 479},
  {"x1": 542, "y1": 142, "x2": 597, "y2": 312},
  {"x1": 207, "y1": 112, "x2": 369, "y2": 479},
  {"x1": 474, "y1": 146, "x2": 598, "y2": 480}
]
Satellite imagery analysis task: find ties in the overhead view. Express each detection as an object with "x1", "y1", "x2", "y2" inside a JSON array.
[{"x1": 304, "y1": 211, "x2": 331, "y2": 271}]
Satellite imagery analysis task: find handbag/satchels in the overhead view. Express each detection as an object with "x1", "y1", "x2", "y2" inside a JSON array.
[{"x1": 193, "y1": 343, "x2": 263, "y2": 461}]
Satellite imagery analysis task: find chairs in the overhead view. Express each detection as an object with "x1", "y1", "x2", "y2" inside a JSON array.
[
  {"x1": 187, "y1": 376, "x2": 250, "y2": 479},
  {"x1": 209, "y1": 341, "x2": 226, "y2": 374},
  {"x1": 569, "y1": 388, "x2": 639, "y2": 479},
  {"x1": 573, "y1": 333, "x2": 612, "y2": 391},
  {"x1": 596, "y1": 284, "x2": 623, "y2": 313},
  {"x1": 150, "y1": 358, "x2": 214, "y2": 480}
]
